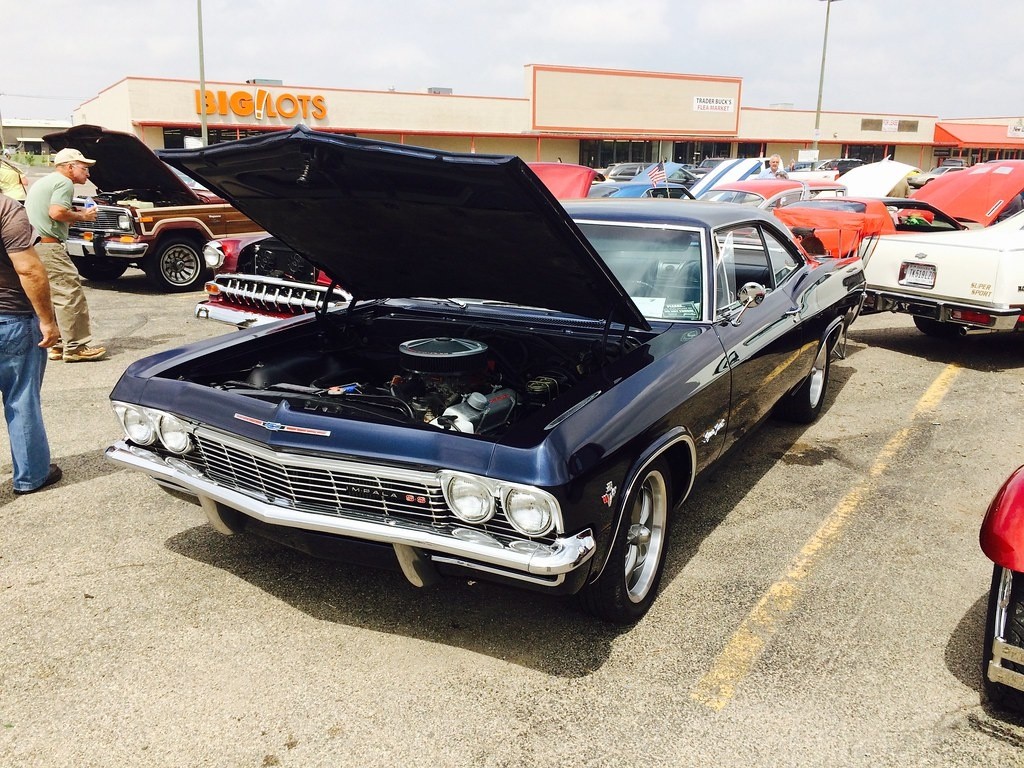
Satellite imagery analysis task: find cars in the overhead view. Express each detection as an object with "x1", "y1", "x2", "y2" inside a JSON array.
[
  {"x1": 22, "y1": 124, "x2": 1024, "y2": 353},
  {"x1": 106, "y1": 124, "x2": 867, "y2": 622}
]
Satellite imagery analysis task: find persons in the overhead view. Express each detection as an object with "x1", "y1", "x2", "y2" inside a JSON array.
[
  {"x1": 0, "y1": 191, "x2": 63, "y2": 495},
  {"x1": 791, "y1": 226, "x2": 826, "y2": 256},
  {"x1": 775, "y1": 169, "x2": 791, "y2": 205},
  {"x1": 888, "y1": 174, "x2": 911, "y2": 198},
  {"x1": 996, "y1": 194, "x2": 1024, "y2": 223},
  {"x1": 24, "y1": 148, "x2": 107, "y2": 363},
  {"x1": 756, "y1": 152, "x2": 784, "y2": 206},
  {"x1": 788, "y1": 158, "x2": 796, "y2": 171}
]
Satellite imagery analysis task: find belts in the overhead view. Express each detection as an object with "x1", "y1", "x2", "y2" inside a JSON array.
[{"x1": 38, "y1": 236, "x2": 60, "y2": 242}]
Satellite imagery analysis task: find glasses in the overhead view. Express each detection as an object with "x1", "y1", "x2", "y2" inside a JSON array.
[{"x1": 76, "y1": 166, "x2": 90, "y2": 174}]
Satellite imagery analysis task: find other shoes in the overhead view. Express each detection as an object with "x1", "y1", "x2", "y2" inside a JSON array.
[
  {"x1": 64, "y1": 345, "x2": 106, "y2": 361},
  {"x1": 48, "y1": 347, "x2": 63, "y2": 360},
  {"x1": 14, "y1": 464, "x2": 62, "y2": 494}
]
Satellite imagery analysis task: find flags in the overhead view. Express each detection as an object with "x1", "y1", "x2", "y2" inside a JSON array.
[{"x1": 647, "y1": 160, "x2": 666, "y2": 188}]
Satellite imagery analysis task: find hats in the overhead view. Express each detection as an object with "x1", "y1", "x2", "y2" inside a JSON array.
[{"x1": 54, "y1": 147, "x2": 96, "y2": 166}]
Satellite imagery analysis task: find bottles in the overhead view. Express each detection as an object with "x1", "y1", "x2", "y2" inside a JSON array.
[{"x1": 84, "y1": 195, "x2": 94, "y2": 210}]
